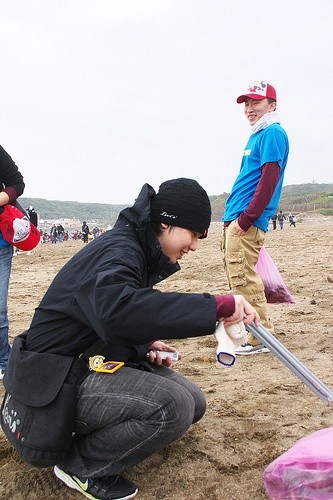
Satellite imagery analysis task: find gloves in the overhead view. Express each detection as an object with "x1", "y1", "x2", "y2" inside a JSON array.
[{"x1": 215, "y1": 320, "x2": 248, "y2": 366}]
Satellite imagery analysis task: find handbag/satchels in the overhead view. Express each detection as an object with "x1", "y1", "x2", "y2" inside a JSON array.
[
  {"x1": 254, "y1": 245, "x2": 297, "y2": 304},
  {"x1": 0, "y1": 330, "x2": 78, "y2": 468},
  {"x1": 262, "y1": 427, "x2": 333, "y2": 500}
]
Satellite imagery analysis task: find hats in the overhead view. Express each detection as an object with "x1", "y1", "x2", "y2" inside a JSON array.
[
  {"x1": 236, "y1": 81, "x2": 277, "y2": 104},
  {"x1": 150, "y1": 178, "x2": 212, "y2": 239},
  {"x1": 0, "y1": 199, "x2": 40, "y2": 251}
]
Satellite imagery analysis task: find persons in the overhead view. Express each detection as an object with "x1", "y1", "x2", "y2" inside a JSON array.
[
  {"x1": 221, "y1": 81, "x2": 290, "y2": 356},
  {"x1": 272, "y1": 210, "x2": 297, "y2": 230},
  {"x1": 3, "y1": 178, "x2": 260, "y2": 500},
  {"x1": 13, "y1": 205, "x2": 111, "y2": 256},
  {"x1": 0, "y1": 145, "x2": 25, "y2": 377}
]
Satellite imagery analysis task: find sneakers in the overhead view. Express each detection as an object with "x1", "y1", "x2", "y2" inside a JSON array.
[
  {"x1": 53, "y1": 464, "x2": 138, "y2": 500},
  {"x1": 235, "y1": 341, "x2": 270, "y2": 355}
]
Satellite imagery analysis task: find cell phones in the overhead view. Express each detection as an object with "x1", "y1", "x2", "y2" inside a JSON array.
[{"x1": 146, "y1": 351, "x2": 178, "y2": 361}]
